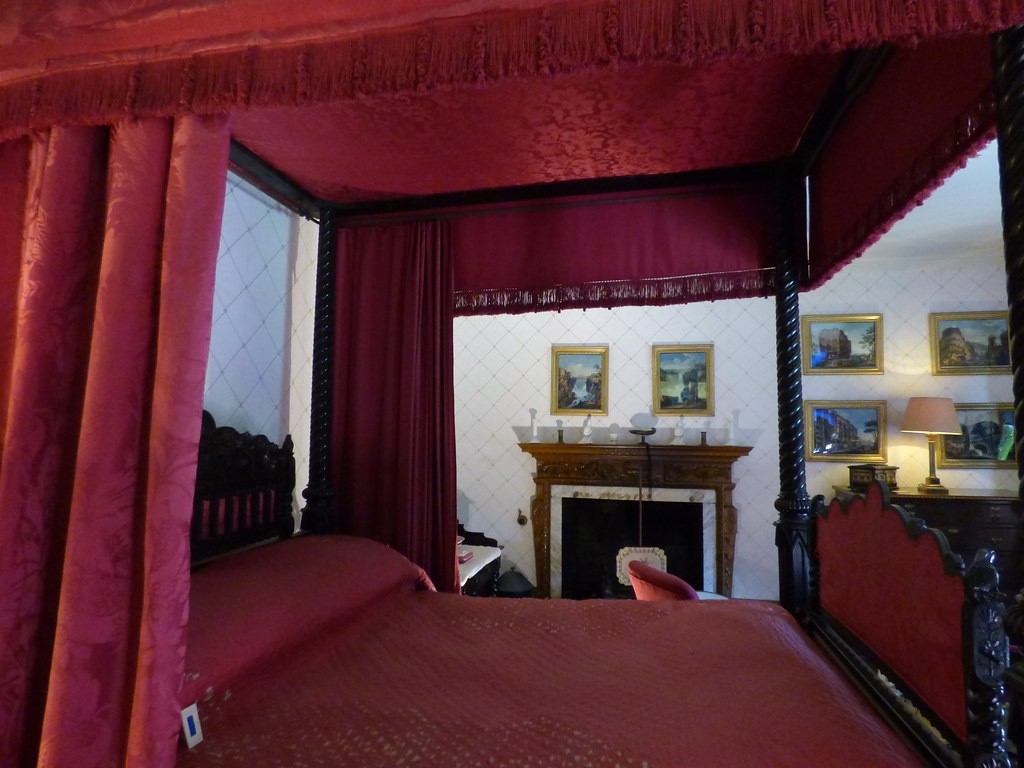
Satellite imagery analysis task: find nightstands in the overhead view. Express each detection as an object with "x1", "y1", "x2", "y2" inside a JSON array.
[{"x1": 299, "y1": 482, "x2": 505, "y2": 597}]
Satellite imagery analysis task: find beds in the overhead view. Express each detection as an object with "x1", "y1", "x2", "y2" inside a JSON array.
[{"x1": 0, "y1": 0, "x2": 1024, "y2": 768}]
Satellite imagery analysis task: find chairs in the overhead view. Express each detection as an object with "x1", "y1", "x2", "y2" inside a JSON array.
[{"x1": 627, "y1": 560, "x2": 728, "y2": 602}]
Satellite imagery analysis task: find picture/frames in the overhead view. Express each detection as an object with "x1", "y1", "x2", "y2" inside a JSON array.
[
  {"x1": 652, "y1": 343, "x2": 715, "y2": 418},
  {"x1": 935, "y1": 402, "x2": 1019, "y2": 470},
  {"x1": 927, "y1": 309, "x2": 1012, "y2": 377},
  {"x1": 802, "y1": 398, "x2": 889, "y2": 463},
  {"x1": 551, "y1": 346, "x2": 610, "y2": 416},
  {"x1": 801, "y1": 314, "x2": 885, "y2": 375}
]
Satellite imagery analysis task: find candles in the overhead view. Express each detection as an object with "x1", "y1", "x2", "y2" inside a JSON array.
[{"x1": 532, "y1": 415, "x2": 537, "y2": 437}]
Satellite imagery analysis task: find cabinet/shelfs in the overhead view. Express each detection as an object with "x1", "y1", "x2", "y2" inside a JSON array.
[{"x1": 833, "y1": 485, "x2": 1024, "y2": 646}]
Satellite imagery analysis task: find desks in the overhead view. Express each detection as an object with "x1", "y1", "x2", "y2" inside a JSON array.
[{"x1": 517, "y1": 441, "x2": 754, "y2": 599}]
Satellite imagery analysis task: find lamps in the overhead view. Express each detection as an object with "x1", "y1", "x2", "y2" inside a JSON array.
[{"x1": 899, "y1": 396, "x2": 962, "y2": 495}]
